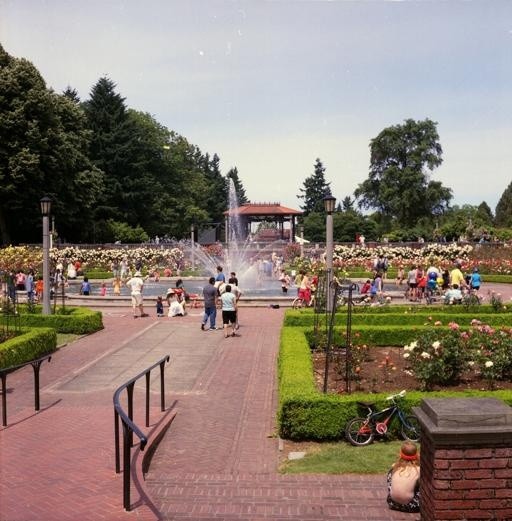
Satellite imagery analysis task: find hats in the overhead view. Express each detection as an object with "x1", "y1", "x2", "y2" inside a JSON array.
[
  {"x1": 133, "y1": 272, "x2": 143, "y2": 277},
  {"x1": 166, "y1": 288, "x2": 175, "y2": 295}
]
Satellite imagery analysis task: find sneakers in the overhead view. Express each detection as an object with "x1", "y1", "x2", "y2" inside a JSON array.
[{"x1": 200, "y1": 322, "x2": 239, "y2": 338}]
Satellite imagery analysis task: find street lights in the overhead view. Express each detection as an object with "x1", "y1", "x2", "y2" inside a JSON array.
[
  {"x1": 39, "y1": 195, "x2": 51, "y2": 317},
  {"x1": 322, "y1": 192, "x2": 337, "y2": 315},
  {"x1": 298, "y1": 223, "x2": 305, "y2": 258},
  {"x1": 190, "y1": 222, "x2": 195, "y2": 271}
]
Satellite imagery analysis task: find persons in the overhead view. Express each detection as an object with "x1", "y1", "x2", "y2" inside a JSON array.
[
  {"x1": 220, "y1": 277, "x2": 242, "y2": 331},
  {"x1": 54, "y1": 258, "x2": 82, "y2": 287},
  {"x1": 80, "y1": 275, "x2": 122, "y2": 295},
  {"x1": 386, "y1": 441, "x2": 424, "y2": 514},
  {"x1": 200, "y1": 277, "x2": 219, "y2": 331},
  {"x1": 219, "y1": 285, "x2": 240, "y2": 338},
  {"x1": 112, "y1": 256, "x2": 184, "y2": 281},
  {"x1": 279, "y1": 269, "x2": 382, "y2": 305},
  {"x1": 373, "y1": 254, "x2": 388, "y2": 279},
  {"x1": 125, "y1": 271, "x2": 149, "y2": 319},
  {"x1": 1, "y1": 268, "x2": 43, "y2": 303},
  {"x1": 397, "y1": 261, "x2": 482, "y2": 304},
  {"x1": 175, "y1": 266, "x2": 238, "y2": 305},
  {"x1": 166, "y1": 288, "x2": 185, "y2": 317},
  {"x1": 156, "y1": 295, "x2": 166, "y2": 317}
]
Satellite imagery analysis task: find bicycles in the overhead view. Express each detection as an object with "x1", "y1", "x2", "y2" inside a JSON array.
[
  {"x1": 292, "y1": 293, "x2": 315, "y2": 309},
  {"x1": 345, "y1": 388, "x2": 423, "y2": 447},
  {"x1": 405, "y1": 280, "x2": 481, "y2": 304}
]
